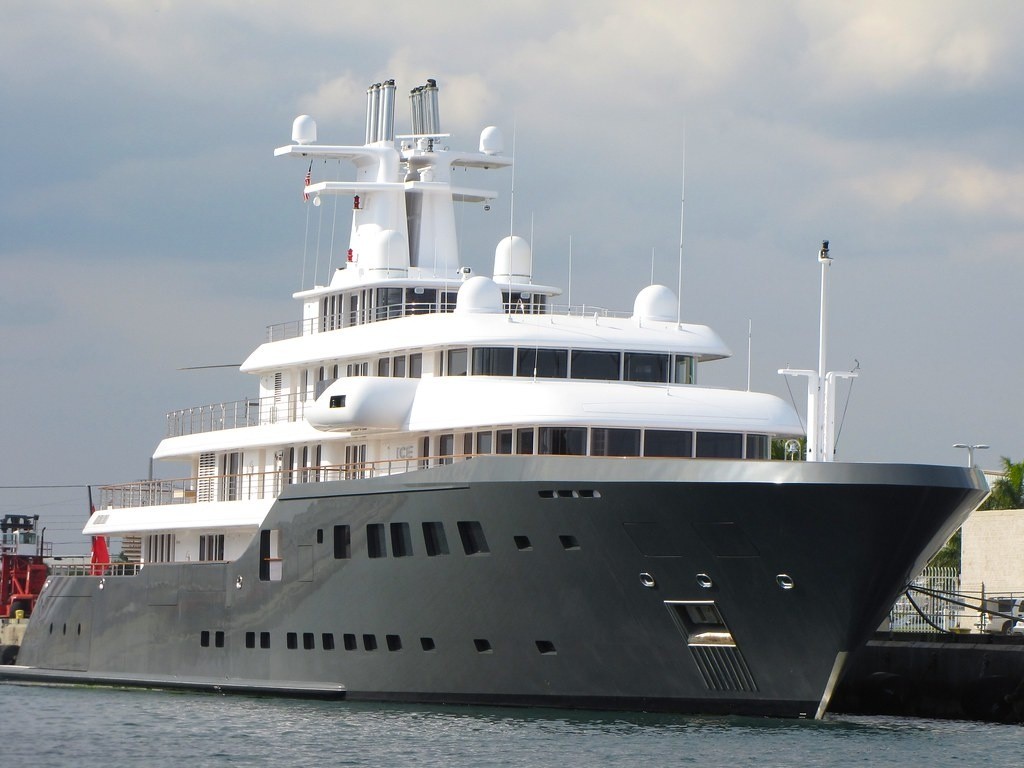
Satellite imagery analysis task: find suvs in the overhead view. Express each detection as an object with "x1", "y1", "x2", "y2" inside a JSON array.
[{"x1": 979, "y1": 596, "x2": 1024, "y2": 637}]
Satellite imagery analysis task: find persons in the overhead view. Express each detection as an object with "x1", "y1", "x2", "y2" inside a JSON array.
[{"x1": 12, "y1": 527, "x2": 36, "y2": 553}]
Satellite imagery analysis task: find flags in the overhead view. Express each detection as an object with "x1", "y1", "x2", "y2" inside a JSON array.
[
  {"x1": 89, "y1": 505, "x2": 109, "y2": 576},
  {"x1": 303, "y1": 165, "x2": 312, "y2": 202}
]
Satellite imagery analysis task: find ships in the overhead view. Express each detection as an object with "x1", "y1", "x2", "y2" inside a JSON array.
[{"x1": 0, "y1": 76, "x2": 996, "y2": 723}]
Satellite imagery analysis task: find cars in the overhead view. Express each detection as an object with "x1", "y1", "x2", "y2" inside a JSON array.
[
  {"x1": 891, "y1": 614, "x2": 955, "y2": 629},
  {"x1": 1013, "y1": 618, "x2": 1024, "y2": 637}
]
